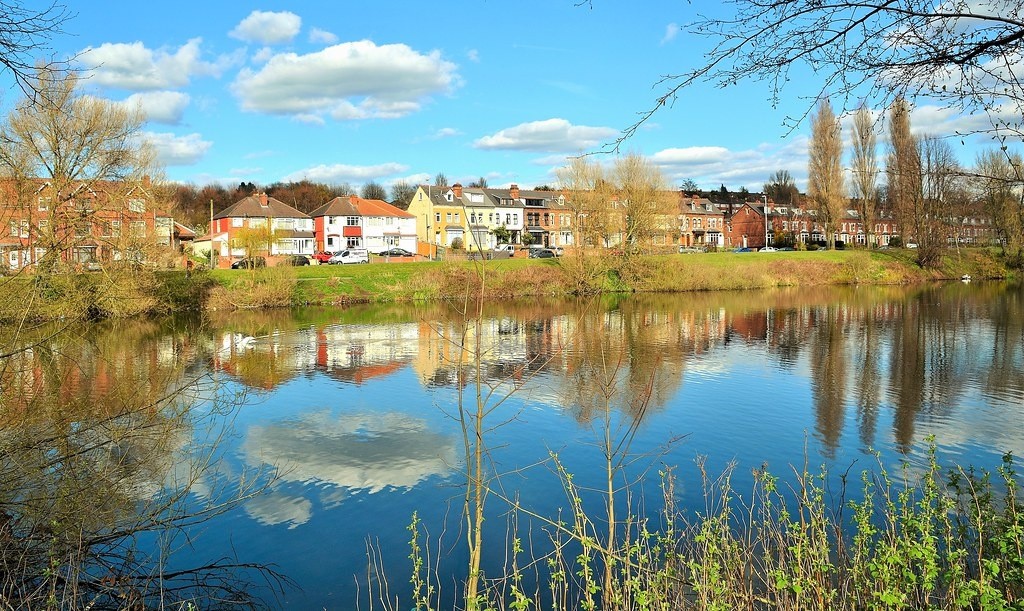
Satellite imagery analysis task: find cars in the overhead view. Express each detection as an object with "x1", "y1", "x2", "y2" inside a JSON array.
[
  {"x1": 380, "y1": 248, "x2": 413, "y2": 256},
  {"x1": 312, "y1": 251, "x2": 332, "y2": 263},
  {"x1": 759, "y1": 247, "x2": 777, "y2": 252},
  {"x1": 531, "y1": 250, "x2": 554, "y2": 259},
  {"x1": 277, "y1": 256, "x2": 310, "y2": 267}
]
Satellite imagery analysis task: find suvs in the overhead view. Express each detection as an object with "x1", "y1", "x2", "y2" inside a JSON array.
[{"x1": 231, "y1": 256, "x2": 267, "y2": 269}]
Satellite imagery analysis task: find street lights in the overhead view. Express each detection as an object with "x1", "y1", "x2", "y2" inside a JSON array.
[
  {"x1": 762, "y1": 195, "x2": 767, "y2": 253},
  {"x1": 426, "y1": 176, "x2": 432, "y2": 262}
]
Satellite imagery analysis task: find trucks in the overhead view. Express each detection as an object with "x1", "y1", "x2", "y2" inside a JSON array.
[{"x1": 328, "y1": 249, "x2": 368, "y2": 264}]
